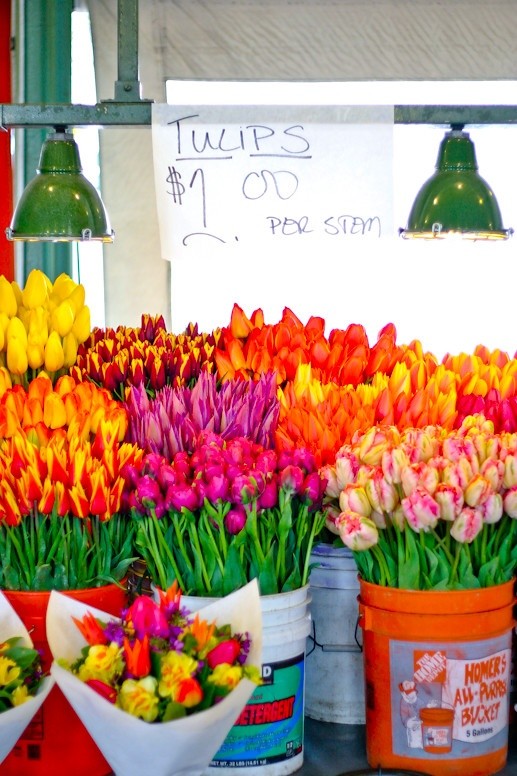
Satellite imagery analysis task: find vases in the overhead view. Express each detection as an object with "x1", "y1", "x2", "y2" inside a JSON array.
[{"x1": 126, "y1": 554, "x2": 154, "y2": 603}]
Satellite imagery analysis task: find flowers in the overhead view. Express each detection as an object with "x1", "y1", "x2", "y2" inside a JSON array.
[{"x1": 0, "y1": 271, "x2": 517, "y2": 776}]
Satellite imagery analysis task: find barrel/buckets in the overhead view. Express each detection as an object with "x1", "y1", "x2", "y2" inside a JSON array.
[
  {"x1": 127, "y1": 554, "x2": 154, "y2": 598},
  {"x1": 353, "y1": 573, "x2": 516, "y2": 774},
  {"x1": 148, "y1": 582, "x2": 313, "y2": 776},
  {"x1": 309, "y1": 542, "x2": 364, "y2": 726},
  {"x1": 0, "y1": 577, "x2": 129, "y2": 775}
]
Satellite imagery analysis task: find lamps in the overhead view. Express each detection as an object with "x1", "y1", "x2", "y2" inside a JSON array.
[
  {"x1": 7, "y1": 125, "x2": 115, "y2": 241},
  {"x1": 399, "y1": 125, "x2": 513, "y2": 240}
]
